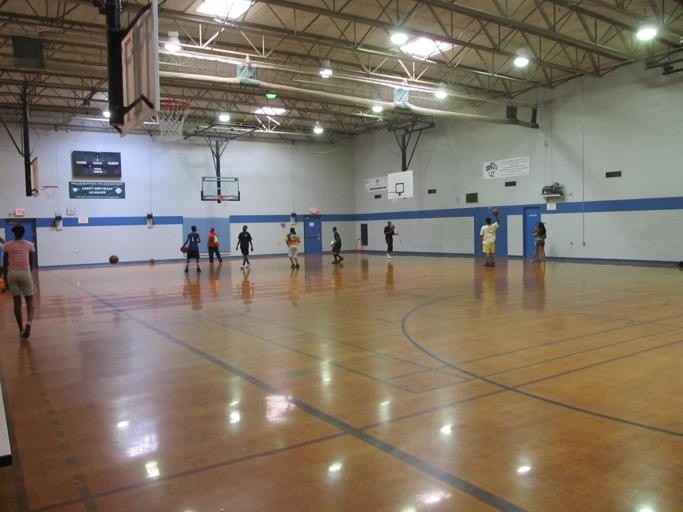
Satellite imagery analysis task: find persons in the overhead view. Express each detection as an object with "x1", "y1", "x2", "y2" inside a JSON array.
[
  {"x1": 1, "y1": 225, "x2": 41, "y2": 340},
  {"x1": 205, "y1": 227, "x2": 222, "y2": 263},
  {"x1": 330, "y1": 226, "x2": 344, "y2": 264},
  {"x1": 284, "y1": 227, "x2": 300, "y2": 269},
  {"x1": 479, "y1": 214, "x2": 501, "y2": 266},
  {"x1": 235, "y1": 225, "x2": 253, "y2": 268},
  {"x1": 383, "y1": 220, "x2": 399, "y2": 260},
  {"x1": 528, "y1": 222, "x2": 546, "y2": 264},
  {"x1": 180, "y1": 225, "x2": 201, "y2": 272}
]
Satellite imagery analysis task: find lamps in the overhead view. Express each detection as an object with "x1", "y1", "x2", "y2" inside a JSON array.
[{"x1": 163, "y1": 30, "x2": 181, "y2": 52}]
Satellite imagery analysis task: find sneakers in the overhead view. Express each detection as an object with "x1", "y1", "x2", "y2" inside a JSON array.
[
  {"x1": 22, "y1": 322, "x2": 32, "y2": 339},
  {"x1": 184, "y1": 253, "x2": 394, "y2": 273}
]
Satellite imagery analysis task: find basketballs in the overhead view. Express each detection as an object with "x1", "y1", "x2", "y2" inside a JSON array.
[
  {"x1": 109, "y1": 255, "x2": 118, "y2": 263},
  {"x1": 492, "y1": 208, "x2": 499, "y2": 215},
  {"x1": 181, "y1": 246, "x2": 187, "y2": 253},
  {"x1": 390, "y1": 225, "x2": 395, "y2": 229}
]
